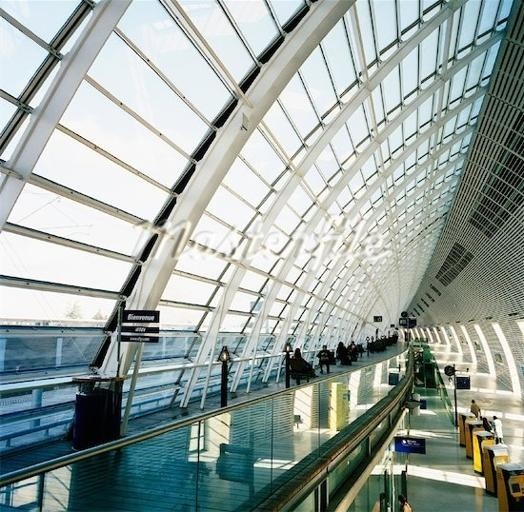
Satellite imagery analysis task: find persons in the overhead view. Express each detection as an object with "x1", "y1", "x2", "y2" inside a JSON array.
[
  {"x1": 370, "y1": 492, "x2": 386, "y2": 511},
  {"x1": 492, "y1": 414, "x2": 504, "y2": 444},
  {"x1": 470, "y1": 398, "x2": 481, "y2": 418},
  {"x1": 464, "y1": 367, "x2": 471, "y2": 380},
  {"x1": 335, "y1": 340, "x2": 352, "y2": 366},
  {"x1": 316, "y1": 344, "x2": 333, "y2": 374},
  {"x1": 398, "y1": 493, "x2": 413, "y2": 512},
  {"x1": 345, "y1": 341, "x2": 358, "y2": 362},
  {"x1": 482, "y1": 417, "x2": 492, "y2": 438},
  {"x1": 293, "y1": 348, "x2": 318, "y2": 378}
]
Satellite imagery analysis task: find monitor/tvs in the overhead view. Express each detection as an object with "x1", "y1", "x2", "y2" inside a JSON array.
[
  {"x1": 456, "y1": 377, "x2": 471, "y2": 389},
  {"x1": 393, "y1": 436, "x2": 427, "y2": 455},
  {"x1": 218, "y1": 443, "x2": 252, "y2": 484},
  {"x1": 389, "y1": 373, "x2": 399, "y2": 385}
]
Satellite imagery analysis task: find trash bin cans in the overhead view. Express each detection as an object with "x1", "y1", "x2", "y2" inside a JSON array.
[
  {"x1": 419, "y1": 399, "x2": 426, "y2": 409},
  {"x1": 72, "y1": 376, "x2": 124, "y2": 452}
]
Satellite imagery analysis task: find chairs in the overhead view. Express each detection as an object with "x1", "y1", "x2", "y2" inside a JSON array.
[{"x1": 289, "y1": 344, "x2": 367, "y2": 384}]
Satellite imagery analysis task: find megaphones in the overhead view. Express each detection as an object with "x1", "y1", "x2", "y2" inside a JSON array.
[{"x1": 401, "y1": 311, "x2": 408, "y2": 317}]
